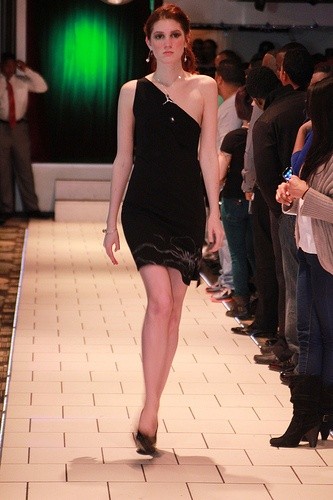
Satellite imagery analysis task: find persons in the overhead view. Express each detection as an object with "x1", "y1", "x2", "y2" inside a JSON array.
[
  {"x1": 0, "y1": 50, "x2": 52, "y2": 223},
  {"x1": 103, "y1": 3, "x2": 229, "y2": 454},
  {"x1": 190, "y1": 36, "x2": 333, "y2": 448}
]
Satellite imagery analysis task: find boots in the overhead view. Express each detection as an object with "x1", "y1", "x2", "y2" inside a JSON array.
[{"x1": 270, "y1": 376, "x2": 333, "y2": 449}]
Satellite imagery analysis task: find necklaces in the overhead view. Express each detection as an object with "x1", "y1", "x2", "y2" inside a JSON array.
[
  {"x1": 242, "y1": 125, "x2": 247, "y2": 128},
  {"x1": 153, "y1": 67, "x2": 187, "y2": 106}
]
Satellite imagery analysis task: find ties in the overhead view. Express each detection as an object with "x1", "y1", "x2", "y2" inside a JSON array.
[{"x1": 6, "y1": 78, "x2": 16, "y2": 129}]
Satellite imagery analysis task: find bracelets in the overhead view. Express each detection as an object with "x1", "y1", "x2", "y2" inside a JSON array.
[
  {"x1": 103, "y1": 228, "x2": 118, "y2": 234},
  {"x1": 302, "y1": 189, "x2": 309, "y2": 200}
]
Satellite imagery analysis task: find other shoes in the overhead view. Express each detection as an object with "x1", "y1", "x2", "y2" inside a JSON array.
[
  {"x1": 225, "y1": 304, "x2": 255, "y2": 320},
  {"x1": 206, "y1": 282, "x2": 222, "y2": 292},
  {"x1": 231, "y1": 316, "x2": 298, "y2": 383},
  {"x1": 210, "y1": 287, "x2": 235, "y2": 303}
]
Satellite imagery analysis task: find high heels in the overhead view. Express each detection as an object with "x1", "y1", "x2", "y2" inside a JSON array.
[{"x1": 135, "y1": 407, "x2": 159, "y2": 456}]
